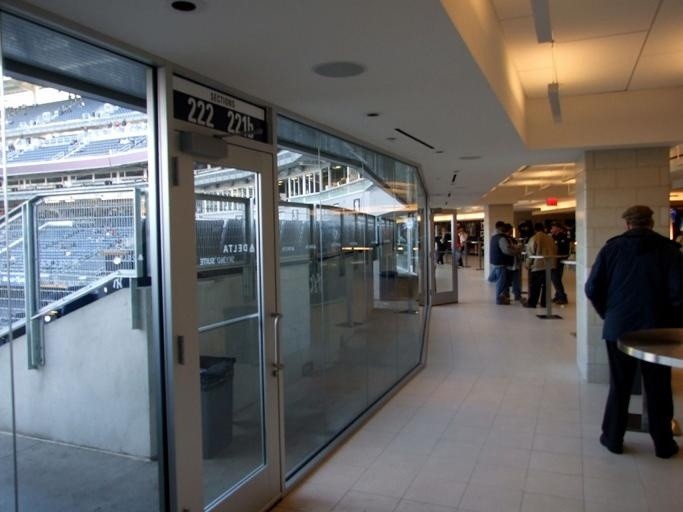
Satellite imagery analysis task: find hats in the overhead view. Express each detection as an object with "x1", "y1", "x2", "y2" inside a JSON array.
[
  {"x1": 621, "y1": 205, "x2": 654, "y2": 221},
  {"x1": 548, "y1": 220, "x2": 561, "y2": 227}
]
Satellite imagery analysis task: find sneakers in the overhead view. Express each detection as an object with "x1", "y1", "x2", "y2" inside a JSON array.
[
  {"x1": 600, "y1": 434, "x2": 624, "y2": 454},
  {"x1": 655, "y1": 441, "x2": 680, "y2": 459},
  {"x1": 496, "y1": 293, "x2": 569, "y2": 308}
]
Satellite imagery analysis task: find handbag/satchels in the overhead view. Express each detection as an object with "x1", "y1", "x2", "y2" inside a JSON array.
[{"x1": 488, "y1": 267, "x2": 501, "y2": 283}]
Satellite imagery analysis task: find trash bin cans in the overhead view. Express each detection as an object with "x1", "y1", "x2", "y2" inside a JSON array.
[
  {"x1": 380, "y1": 271, "x2": 398, "y2": 300},
  {"x1": 199, "y1": 356, "x2": 236, "y2": 460}
]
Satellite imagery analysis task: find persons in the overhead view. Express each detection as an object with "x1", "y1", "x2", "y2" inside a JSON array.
[
  {"x1": 585, "y1": 206, "x2": 683, "y2": 459},
  {"x1": 435, "y1": 221, "x2": 570, "y2": 308}
]
